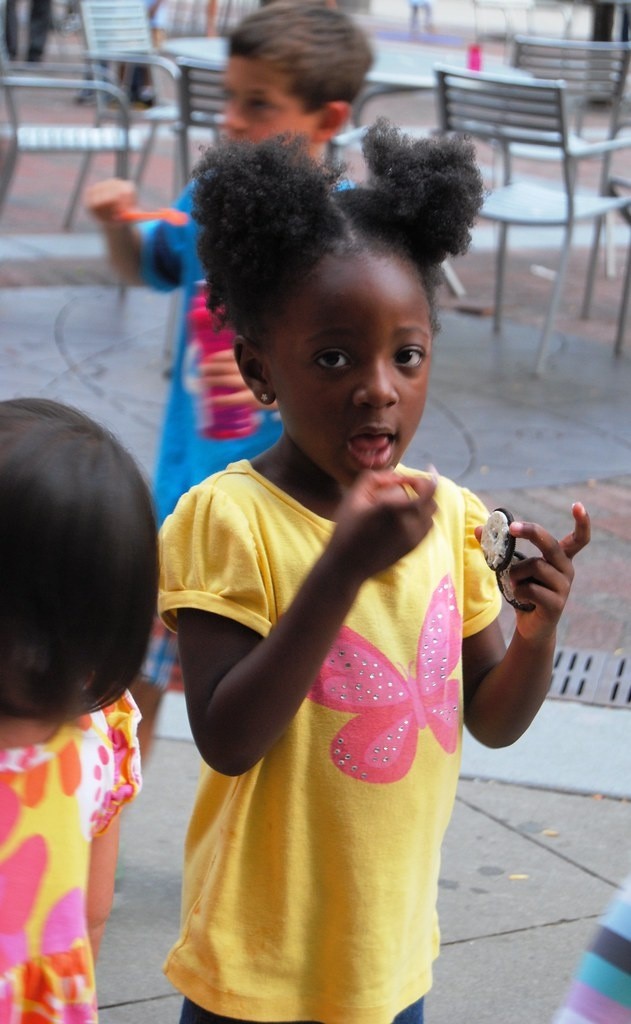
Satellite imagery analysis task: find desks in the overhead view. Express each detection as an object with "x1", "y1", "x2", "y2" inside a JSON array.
[{"x1": 161, "y1": 30, "x2": 533, "y2": 134}]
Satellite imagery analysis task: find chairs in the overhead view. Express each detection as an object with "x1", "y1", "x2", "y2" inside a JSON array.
[{"x1": 0, "y1": 0, "x2": 631, "y2": 380}]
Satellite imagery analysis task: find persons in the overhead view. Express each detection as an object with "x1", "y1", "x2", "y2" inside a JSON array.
[
  {"x1": 158, "y1": 120, "x2": 591, "y2": 1024},
  {"x1": 0, "y1": 398, "x2": 159, "y2": 1024},
  {"x1": 86, "y1": 0, "x2": 375, "y2": 761}
]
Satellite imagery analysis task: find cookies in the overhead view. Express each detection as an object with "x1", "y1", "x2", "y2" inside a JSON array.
[
  {"x1": 496, "y1": 551, "x2": 536, "y2": 612},
  {"x1": 480, "y1": 507, "x2": 516, "y2": 572}
]
